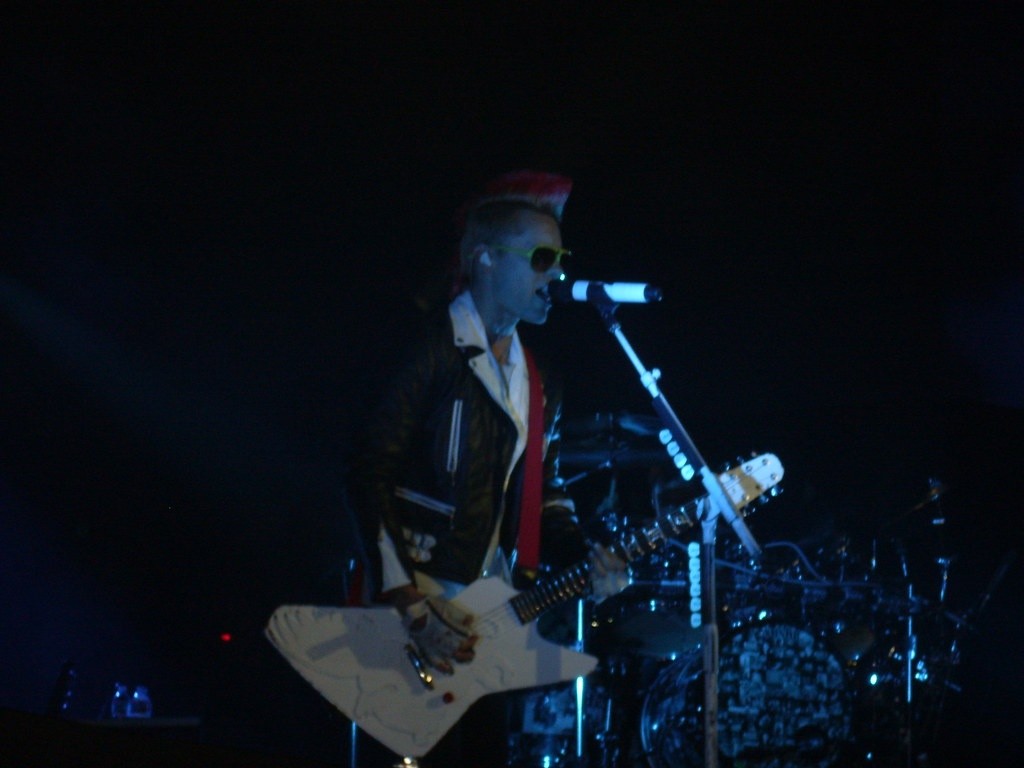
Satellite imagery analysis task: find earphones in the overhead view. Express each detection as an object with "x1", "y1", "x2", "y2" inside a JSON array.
[{"x1": 480, "y1": 252, "x2": 493, "y2": 267}]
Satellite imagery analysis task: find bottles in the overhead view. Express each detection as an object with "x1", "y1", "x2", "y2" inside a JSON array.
[
  {"x1": 111, "y1": 681, "x2": 130, "y2": 719},
  {"x1": 127, "y1": 685, "x2": 152, "y2": 717}
]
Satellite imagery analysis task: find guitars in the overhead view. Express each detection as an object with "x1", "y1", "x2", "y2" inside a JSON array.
[{"x1": 262, "y1": 448, "x2": 793, "y2": 759}]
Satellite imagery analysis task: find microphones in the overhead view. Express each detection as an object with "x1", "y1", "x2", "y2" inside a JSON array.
[{"x1": 547, "y1": 279, "x2": 662, "y2": 306}]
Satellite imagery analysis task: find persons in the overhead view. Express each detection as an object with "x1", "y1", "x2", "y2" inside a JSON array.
[{"x1": 342, "y1": 171, "x2": 573, "y2": 766}]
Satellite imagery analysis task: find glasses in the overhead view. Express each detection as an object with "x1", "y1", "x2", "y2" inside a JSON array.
[{"x1": 470, "y1": 244, "x2": 573, "y2": 275}]
